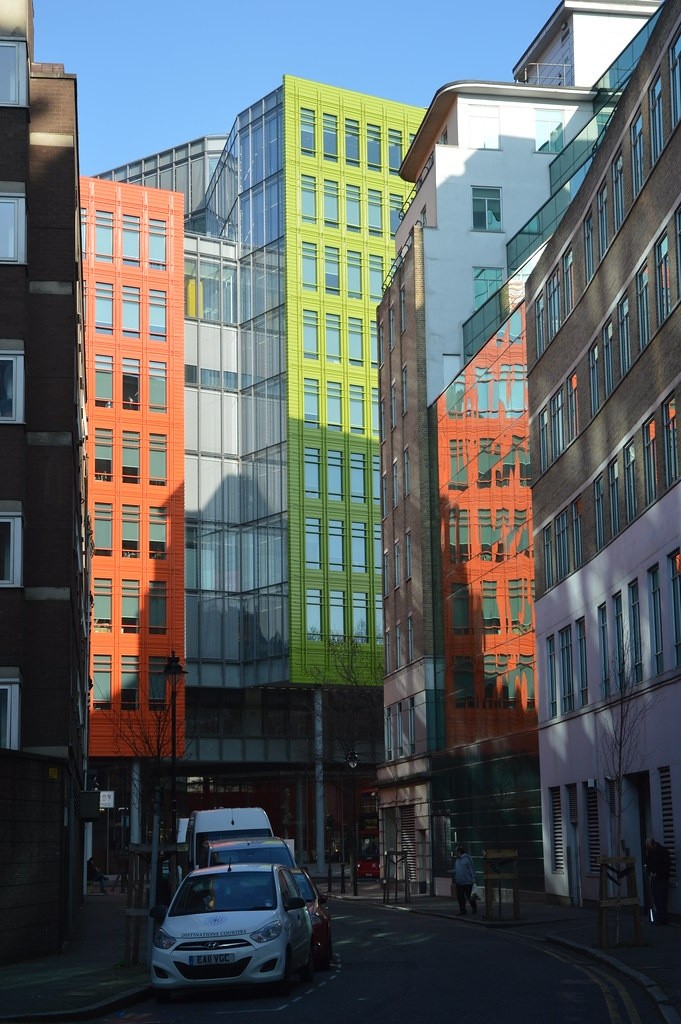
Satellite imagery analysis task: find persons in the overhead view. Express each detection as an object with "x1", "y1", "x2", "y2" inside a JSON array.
[
  {"x1": 447, "y1": 847, "x2": 478, "y2": 915},
  {"x1": 87, "y1": 857, "x2": 108, "y2": 893},
  {"x1": 644, "y1": 838, "x2": 671, "y2": 925}
]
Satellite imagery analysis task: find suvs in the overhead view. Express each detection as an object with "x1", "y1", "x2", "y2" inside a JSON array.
[
  {"x1": 147, "y1": 864, "x2": 317, "y2": 1004},
  {"x1": 199, "y1": 836, "x2": 298, "y2": 895}
]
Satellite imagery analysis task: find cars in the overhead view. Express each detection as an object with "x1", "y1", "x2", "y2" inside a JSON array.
[
  {"x1": 291, "y1": 867, "x2": 334, "y2": 961},
  {"x1": 355, "y1": 854, "x2": 380, "y2": 877}
]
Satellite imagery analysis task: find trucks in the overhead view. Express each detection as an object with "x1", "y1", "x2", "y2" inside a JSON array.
[{"x1": 184, "y1": 804, "x2": 276, "y2": 873}]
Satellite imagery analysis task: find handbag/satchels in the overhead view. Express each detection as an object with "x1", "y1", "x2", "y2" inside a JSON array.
[{"x1": 470, "y1": 881, "x2": 482, "y2": 900}]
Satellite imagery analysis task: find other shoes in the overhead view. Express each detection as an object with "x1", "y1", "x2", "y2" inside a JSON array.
[
  {"x1": 653, "y1": 920, "x2": 669, "y2": 926},
  {"x1": 472, "y1": 909, "x2": 478, "y2": 914},
  {"x1": 456, "y1": 911, "x2": 467, "y2": 916},
  {"x1": 100, "y1": 889, "x2": 108, "y2": 893},
  {"x1": 104, "y1": 877, "x2": 109, "y2": 881}
]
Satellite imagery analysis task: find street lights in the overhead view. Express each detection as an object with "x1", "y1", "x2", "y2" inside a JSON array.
[
  {"x1": 344, "y1": 750, "x2": 359, "y2": 860},
  {"x1": 161, "y1": 648, "x2": 191, "y2": 903}
]
